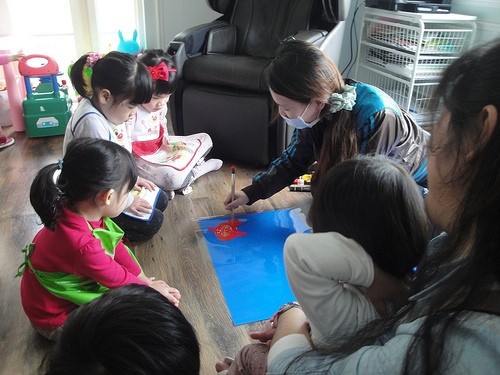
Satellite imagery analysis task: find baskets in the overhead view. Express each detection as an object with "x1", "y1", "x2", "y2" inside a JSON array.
[{"x1": 360, "y1": 15, "x2": 472, "y2": 124}]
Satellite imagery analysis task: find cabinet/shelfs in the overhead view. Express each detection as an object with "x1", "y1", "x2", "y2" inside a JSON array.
[{"x1": 355, "y1": 7, "x2": 476, "y2": 146}]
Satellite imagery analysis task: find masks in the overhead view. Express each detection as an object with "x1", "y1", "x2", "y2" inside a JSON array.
[{"x1": 280, "y1": 99, "x2": 321, "y2": 130}]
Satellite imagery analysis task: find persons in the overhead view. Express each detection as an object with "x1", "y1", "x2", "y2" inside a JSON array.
[
  {"x1": 216, "y1": 155, "x2": 431, "y2": 375},
  {"x1": 131, "y1": 49, "x2": 224, "y2": 200},
  {"x1": 215, "y1": 36, "x2": 500, "y2": 375},
  {"x1": 223, "y1": 41, "x2": 432, "y2": 212},
  {"x1": 62, "y1": 51, "x2": 168, "y2": 242},
  {"x1": 36, "y1": 283, "x2": 200, "y2": 375},
  {"x1": 15, "y1": 137, "x2": 181, "y2": 343}
]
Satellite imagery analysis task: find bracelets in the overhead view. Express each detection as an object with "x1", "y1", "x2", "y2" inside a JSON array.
[{"x1": 270, "y1": 302, "x2": 304, "y2": 328}]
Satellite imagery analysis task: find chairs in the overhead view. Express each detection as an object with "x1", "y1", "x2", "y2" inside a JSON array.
[{"x1": 165, "y1": 0, "x2": 365, "y2": 167}]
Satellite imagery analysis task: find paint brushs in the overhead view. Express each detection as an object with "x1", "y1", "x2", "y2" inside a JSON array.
[{"x1": 229, "y1": 165, "x2": 236, "y2": 222}]
